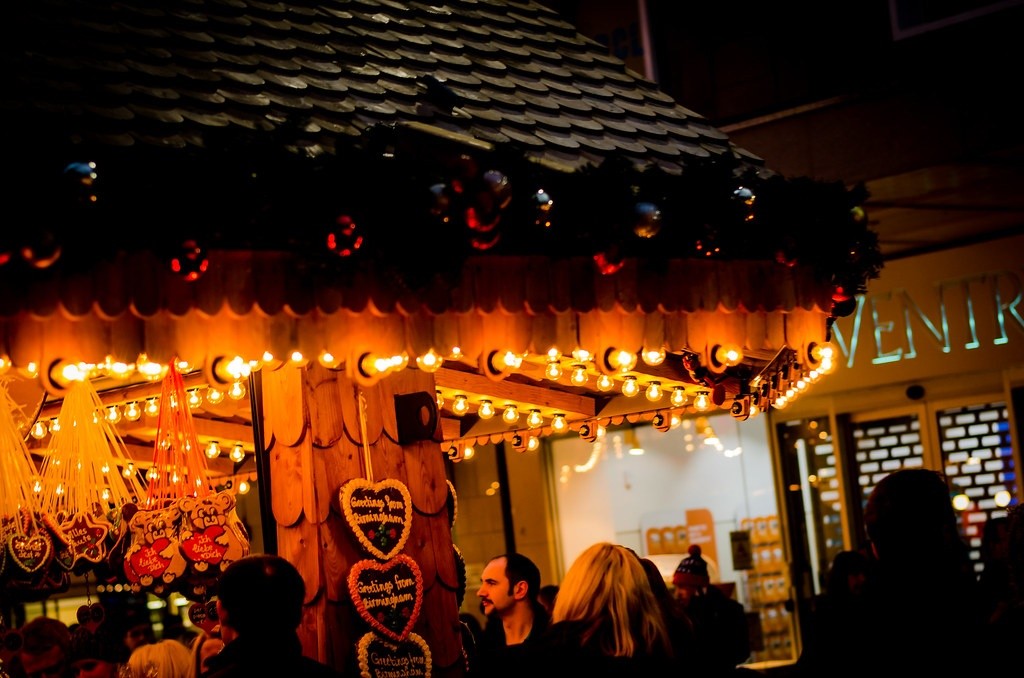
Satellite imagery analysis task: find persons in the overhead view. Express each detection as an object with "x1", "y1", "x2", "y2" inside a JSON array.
[
  {"x1": 0, "y1": 603, "x2": 226, "y2": 678},
  {"x1": 198, "y1": 552, "x2": 347, "y2": 678},
  {"x1": 799, "y1": 468, "x2": 1024, "y2": 678},
  {"x1": 470, "y1": 537, "x2": 754, "y2": 678}
]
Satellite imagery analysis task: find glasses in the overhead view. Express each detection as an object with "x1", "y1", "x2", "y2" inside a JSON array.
[
  {"x1": 69, "y1": 659, "x2": 101, "y2": 675},
  {"x1": 122, "y1": 628, "x2": 148, "y2": 639}
]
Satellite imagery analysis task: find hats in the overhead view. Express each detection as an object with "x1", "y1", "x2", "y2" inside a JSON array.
[
  {"x1": 107, "y1": 603, "x2": 149, "y2": 641},
  {"x1": 216, "y1": 554, "x2": 306, "y2": 631},
  {"x1": 673, "y1": 544, "x2": 709, "y2": 584}
]
